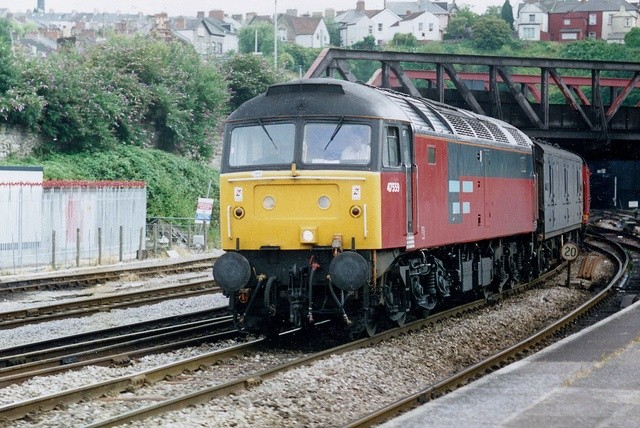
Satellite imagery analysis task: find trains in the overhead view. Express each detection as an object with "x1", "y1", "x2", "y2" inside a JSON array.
[{"x1": 212, "y1": 65, "x2": 593, "y2": 343}]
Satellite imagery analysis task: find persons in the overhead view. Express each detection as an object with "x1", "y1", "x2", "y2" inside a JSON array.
[{"x1": 339, "y1": 130, "x2": 371, "y2": 162}]
